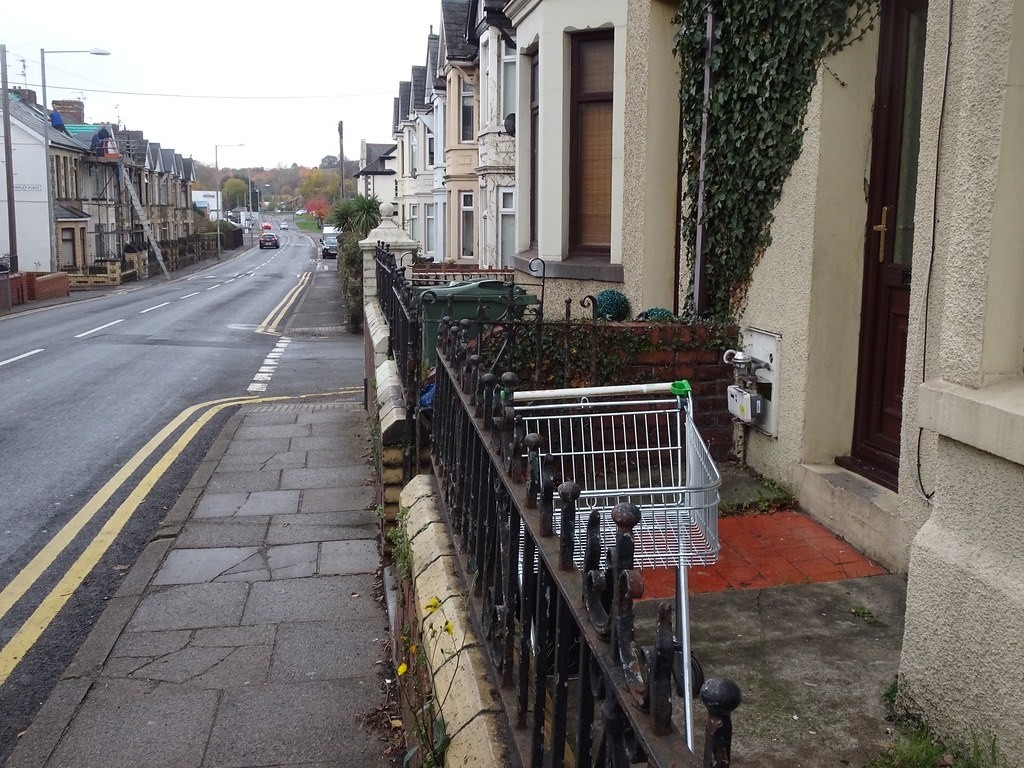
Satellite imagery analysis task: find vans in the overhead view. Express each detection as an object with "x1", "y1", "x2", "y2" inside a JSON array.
[{"x1": 295, "y1": 210, "x2": 307, "y2": 215}]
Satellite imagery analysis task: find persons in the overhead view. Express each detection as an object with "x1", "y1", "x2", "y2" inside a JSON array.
[
  {"x1": 49, "y1": 107, "x2": 73, "y2": 138},
  {"x1": 91, "y1": 125, "x2": 109, "y2": 157}
]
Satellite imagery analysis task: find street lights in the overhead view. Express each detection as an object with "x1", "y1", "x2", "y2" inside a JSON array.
[
  {"x1": 40, "y1": 48, "x2": 111, "y2": 276},
  {"x1": 215, "y1": 144, "x2": 245, "y2": 261},
  {"x1": 257, "y1": 184, "x2": 271, "y2": 233}
]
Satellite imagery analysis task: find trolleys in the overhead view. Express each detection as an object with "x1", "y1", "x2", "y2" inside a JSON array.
[{"x1": 495, "y1": 376, "x2": 722, "y2": 756}]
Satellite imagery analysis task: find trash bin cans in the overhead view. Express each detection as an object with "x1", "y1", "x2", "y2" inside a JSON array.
[{"x1": 413, "y1": 278, "x2": 538, "y2": 377}]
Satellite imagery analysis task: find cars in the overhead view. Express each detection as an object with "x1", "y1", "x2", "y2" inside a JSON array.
[
  {"x1": 280, "y1": 221, "x2": 288, "y2": 230},
  {"x1": 261, "y1": 222, "x2": 273, "y2": 230}
]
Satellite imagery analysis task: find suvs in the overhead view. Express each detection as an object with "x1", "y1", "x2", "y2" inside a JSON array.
[
  {"x1": 259, "y1": 232, "x2": 280, "y2": 249},
  {"x1": 321, "y1": 236, "x2": 339, "y2": 259}
]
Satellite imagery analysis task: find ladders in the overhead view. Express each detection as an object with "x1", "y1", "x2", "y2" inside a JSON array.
[{"x1": 121, "y1": 163, "x2": 170, "y2": 279}]
